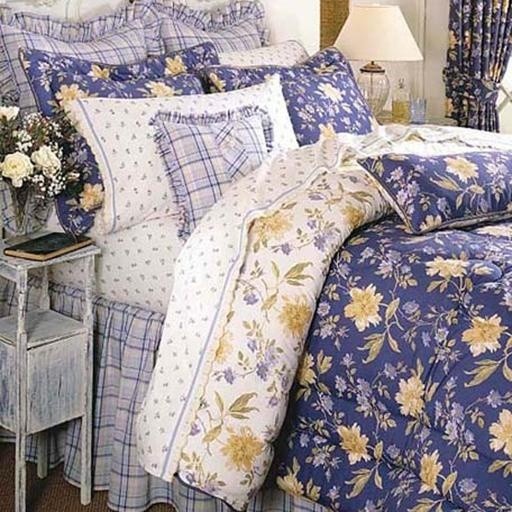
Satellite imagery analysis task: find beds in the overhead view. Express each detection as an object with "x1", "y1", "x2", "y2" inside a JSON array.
[{"x1": 0, "y1": 0, "x2": 512, "y2": 510}]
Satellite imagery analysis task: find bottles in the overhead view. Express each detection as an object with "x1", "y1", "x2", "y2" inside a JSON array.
[{"x1": 392, "y1": 77, "x2": 413, "y2": 126}]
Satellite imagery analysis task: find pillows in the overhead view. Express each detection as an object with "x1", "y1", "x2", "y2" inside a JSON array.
[
  {"x1": 15, "y1": 42, "x2": 223, "y2": 237},
  {"x1": 152, "y1": 2, "x2": 271, "y2": 53},
  {"x1": 200, "y1": 46, "x2": 380, "y2": 147},
  {"x1": 218, "y1": 39, "x2": 312, "y2": 69},
  {"x1": 0, "y1": 1, "x2": 165, "y2": 121},
  {"x1": 64, "y1": 76, "x2": 302, "y2": 239}
]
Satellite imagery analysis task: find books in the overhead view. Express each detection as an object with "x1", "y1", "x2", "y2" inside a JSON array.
[{"x1": 2, "y1": 232, "x2": 94, "y2": 262}]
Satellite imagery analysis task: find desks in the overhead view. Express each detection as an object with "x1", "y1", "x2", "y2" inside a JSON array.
[{"x1": 0, "y1": 244, "x2": 101, "y2": 512}]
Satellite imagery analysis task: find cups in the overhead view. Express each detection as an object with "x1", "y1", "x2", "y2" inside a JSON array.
[{"x1": 410, "y1": 97, "x2": 427, "y2": 125}]
